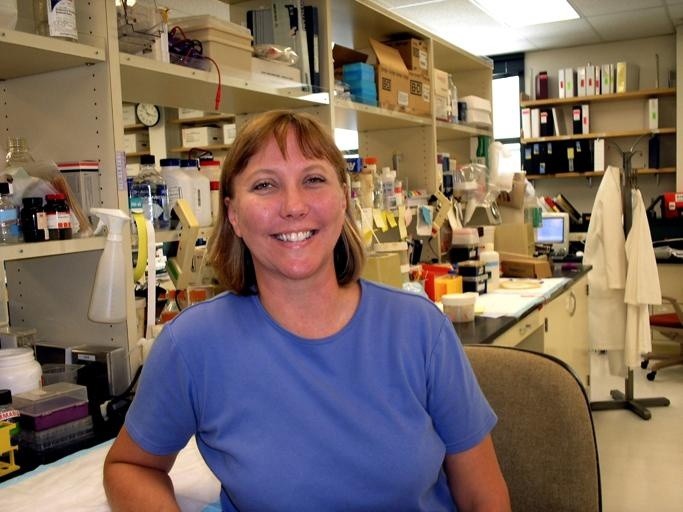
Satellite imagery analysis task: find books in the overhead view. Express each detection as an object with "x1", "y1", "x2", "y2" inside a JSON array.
[{"x1": 534, "y1": 192, "x2": 581, "y2": 226}]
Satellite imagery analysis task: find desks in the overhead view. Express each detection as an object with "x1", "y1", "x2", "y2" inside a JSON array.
[{"x1": 0, "y1": 264, "x2": 595, "y2": 512}]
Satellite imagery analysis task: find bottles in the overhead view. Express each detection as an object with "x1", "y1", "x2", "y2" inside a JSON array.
[
  {"x1": 133, "y1": 154, "x2": 213, "y2": 229},
  {"x1": 447, "y1": 73, "x2": 459, "y2": 124},
  {"x1": 158, "y1": 290, "x2": 183, "y2": 322},
  {"x1": 362, "y1": 149, "x2": 409, "y2": 213},
  {"x1": 34, "y1": 0, "x2": 78, "y2": 40},
  {"x1": 0, "y1": 1, "x2": 18, "y2": 32},
  {"x1": 0, "y1": 390, "x2": 22, "y2": 453},
  {"x1": 0, "y1": 183, "x2": 71, "y2": 245},
  {"x1": 7, "y1": 137, "x2": 35, "y2": 169},
  {"x1": 351, "y1": 190, "x2": 362, "y2": 220}
]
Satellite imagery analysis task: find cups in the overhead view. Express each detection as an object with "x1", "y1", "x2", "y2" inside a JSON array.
[
  {"x1": 442, "y1": 292, "x2": 478, "y2": 324},
  {"x1": 39, "y1": 362, "x2": 86, "y2": 386}
]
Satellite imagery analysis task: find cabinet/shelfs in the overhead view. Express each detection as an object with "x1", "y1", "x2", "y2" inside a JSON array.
[
  {"x1": 520, "y1": 87, "x2": 676, "y2": 188},
  {"x1": 516, "y1": 274, "x2": 591, "y2": 404},
  {"x1": 0, "y1": 0, "x2": 494, "y2": 394}
]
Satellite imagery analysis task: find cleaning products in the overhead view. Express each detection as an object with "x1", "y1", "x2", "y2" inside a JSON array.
[
  {"x1": 160, "y1": 156, "x2": 220, "y2": 226},
  {"x1": 87, "y1": 207, "x2": 132, "y2": 326},
  {"x1": 481, "y1": 242, "x2": 500, "y2": 286}
]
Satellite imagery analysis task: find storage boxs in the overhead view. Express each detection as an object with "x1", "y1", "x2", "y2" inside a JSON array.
[
  {"x1": 332, "y1": 36, "x2": 432, "y2": 117},
  {"x1": 168, "y1": 16, "x2": 302, "y2": 85},
  {"x1": 433, "y1": 69, "x2": 491, "y2": 128}
]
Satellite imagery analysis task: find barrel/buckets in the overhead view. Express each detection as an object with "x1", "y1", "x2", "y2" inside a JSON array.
[{"x1": 0, "y1": 347, "x2": 43, "y2": 398}]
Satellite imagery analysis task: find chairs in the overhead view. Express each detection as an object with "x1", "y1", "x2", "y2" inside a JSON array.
[
  {"x1": 641, "y1": 296, "x2": 683, "y2": 381},
  {"x1": 441, "y1": 343, "x2": 602, "y2": 512}
]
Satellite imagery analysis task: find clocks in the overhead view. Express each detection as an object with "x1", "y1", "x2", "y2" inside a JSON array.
[{"x1": 136, "y1": 102, "x2": 160, "y2": 127}]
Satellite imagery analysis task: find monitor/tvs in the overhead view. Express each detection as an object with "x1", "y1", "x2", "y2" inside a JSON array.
[{"x1": 534, "y1": 212, "x2": 570, "y2": 256}]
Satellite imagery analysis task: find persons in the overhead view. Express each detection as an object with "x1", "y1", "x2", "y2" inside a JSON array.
[{"x1": 99, "y1": 107, "x2": 512, "y2": 511}]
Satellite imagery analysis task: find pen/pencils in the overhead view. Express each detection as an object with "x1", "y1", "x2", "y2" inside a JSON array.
[{"x1": 511, "y1": 279, "x2": 544, "y2": 283}]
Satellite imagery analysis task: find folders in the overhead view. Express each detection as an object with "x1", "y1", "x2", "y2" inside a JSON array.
[
  {"x1": 520, "y1": 139, "x2": 605, "y2": 173},
  {"x1": 246, "y1": 6, "x2": 320, "y2": 93},
  {"x1": 645, "y1": 98, "x2": 658, "y2": 130},
  {"x1": 649, "y1": 139, "x2": 660, "y2": 169},
  {"x1": 521, "y1": 105, "x2": 590, "y2": 139},
  {"x1": 558, "y1": 62, "x2": 639, "y2": 97}
]
